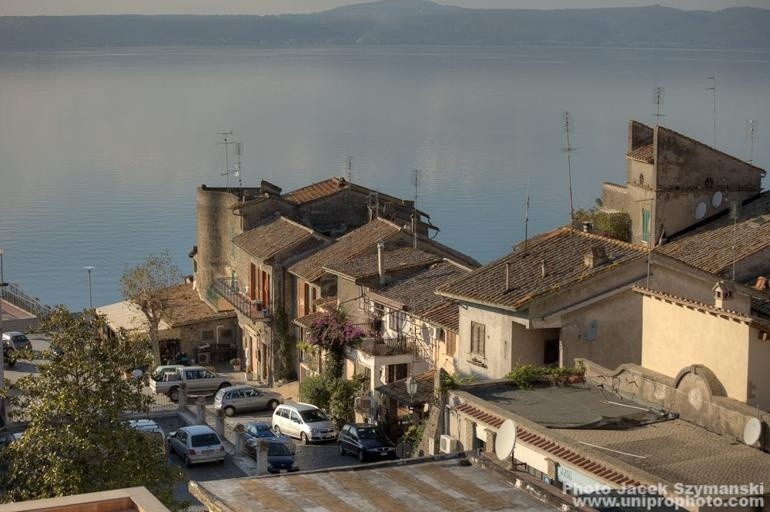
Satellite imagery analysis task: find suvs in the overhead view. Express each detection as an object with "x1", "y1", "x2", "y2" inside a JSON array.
[{"x1": 2, "y1": 335, "x2": 18, "y2": 369}]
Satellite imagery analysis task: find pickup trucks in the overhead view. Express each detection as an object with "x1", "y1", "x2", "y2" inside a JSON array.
[{"x1": 149, "y1": 365, "x2": 234, "y2": 402}]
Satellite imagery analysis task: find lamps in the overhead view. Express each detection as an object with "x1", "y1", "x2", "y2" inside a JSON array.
[{"x1": 404, "y1": 373, "x2": 420, "y2": 402}]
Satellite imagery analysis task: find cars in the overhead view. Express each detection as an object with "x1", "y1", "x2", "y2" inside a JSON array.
[
  {"x1": 335, "y1": 422, "x2": 397, "y2": 461},
  {"x1": 167, "y1": 423, "x2": 227, "y2": 469},
  {"x1": 243, "y1": 436, "x2": 299, "y2": 474},
  {"x1": 2, "y1": 331, "x2": 33, "y2": 354},
  {"x1": 211, "y1": 384, "x2": 285, "y2": 417},
  {"x1": 241, "y1": 419, "x2": 275, "y2": 438}
]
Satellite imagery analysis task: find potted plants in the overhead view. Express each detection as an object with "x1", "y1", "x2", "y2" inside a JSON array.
[
  {"x1": 245, "y1": 367, "x2": 253, "y2": 380},
  {"x1": 230, "y1": 358, "x2": 241, "y2": 371}
]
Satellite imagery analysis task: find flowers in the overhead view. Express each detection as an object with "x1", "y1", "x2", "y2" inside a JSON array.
[{"x1": 307, "y1": 303, "x2": 365, "y2": 352}]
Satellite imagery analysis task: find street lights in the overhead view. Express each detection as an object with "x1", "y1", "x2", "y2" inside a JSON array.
[{"x1": 82, "y1": 265, "x2": 94, "y2": 322}]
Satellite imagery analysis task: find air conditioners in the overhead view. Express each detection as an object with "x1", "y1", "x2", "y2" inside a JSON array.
[
  {"x1": 197, "y1": 351, "x2": 211, "y2": 364},
  {"x1": 439, "y1": 434, "x2": 457, "y2": 455}
]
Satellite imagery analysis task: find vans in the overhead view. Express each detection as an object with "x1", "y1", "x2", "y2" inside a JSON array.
[
  {"x1": 270, "y1": 400, "x2": 339, "y2": 444},
  {"x1": 122, "y1": 417, "x2": 167, "y2": 460}
]
